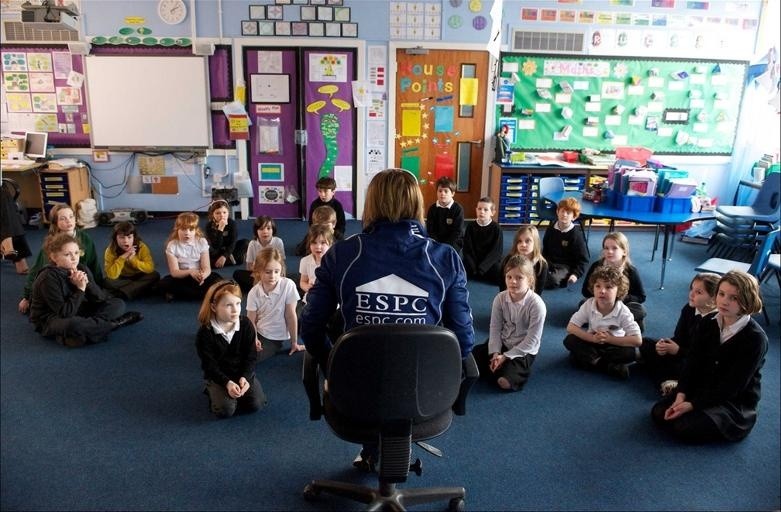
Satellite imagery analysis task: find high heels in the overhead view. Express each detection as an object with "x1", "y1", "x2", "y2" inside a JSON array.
[
  {"x1": 16, "y1": 262, "x2": 30, "y2": 274},
  {"x1": 0, "y1": 243, "x2": 18, "y2": 259}
]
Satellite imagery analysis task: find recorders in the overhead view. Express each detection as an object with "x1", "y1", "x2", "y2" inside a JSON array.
[{"x1": 99, "y1": 207, "x2": 148, "y2": 227}]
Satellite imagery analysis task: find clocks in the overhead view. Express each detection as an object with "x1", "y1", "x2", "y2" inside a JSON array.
[{"x1": 156, "y1": 0, "x2": 189, "y2": 27}]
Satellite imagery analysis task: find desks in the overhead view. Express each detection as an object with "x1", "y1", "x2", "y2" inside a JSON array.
[
  {"x1": 544, "y1": 185, "x2": 695, "y2": 292},
  {"x1": 0, "y1": 157, "x2": 57, "y2": 233}
]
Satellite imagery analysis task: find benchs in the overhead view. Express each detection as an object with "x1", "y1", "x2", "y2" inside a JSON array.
[{"x1": 92, "y1": 148, "x2": 110, "y2": 165}]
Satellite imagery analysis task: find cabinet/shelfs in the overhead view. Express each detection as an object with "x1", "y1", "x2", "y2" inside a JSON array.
[{"x1": 489, "y1": 162, "x2": 689, "y2": 233}]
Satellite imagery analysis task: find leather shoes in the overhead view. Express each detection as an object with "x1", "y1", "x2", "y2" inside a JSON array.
[
  {"x1": 112, "y1": 312, "x2": 144, "y2": 327},
  {"x1": 56, "y1": 335, "x2": 87, "y2": 347}
]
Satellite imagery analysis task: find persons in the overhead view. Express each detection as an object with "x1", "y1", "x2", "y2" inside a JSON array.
[
  {"x1": 427, "y1": 177, "x2": 464, "y2": 257},
  {"x1": 563, "y1": 264, "x2": 643, "y2": 382},
  {"x1": 0, "y1": 184, "x2": 33, "y2": 276},
  {"x1": 470, "y1": 253, "x2": 547, "y2": 393},
  {"x1": 639, "y1": 272, "x2": 723, "y2": 388},
  {"x1": 300, "y1": 168, "x2": 476, "y2": 473},
  {"x1": 650, "y1": 270, "x2": 769, "y2": 446},
  {"x1": 578, "y1": 232, "x2": 647, "y2": 334},
  {"x1": 26, "y1": 232, "x2": 144, "y2": 349},
  {"x1": 460, "y1": 197, "x2": 504, "y2": 280},
  {"x1": 540, "y1": 196, "x2": 589, "y2": 290},
  {"x1": 195, "y1": 176, "x2": 345, "y2": 419},
  {"x1": 18, "y1": 204, "x2": 103, "y2": 314},
  {"x1": 495, "y1": 125, "x2": 509, "y2": 164},
  {"x1": 102, "y1": 222, "x2": 160, "y2": 302},
  {"x1": 156, "y1": 212, "x2": 225, "y2": 303},
  {"x1": 498, "y1": 225, "x2": 549, "y2": 297}
]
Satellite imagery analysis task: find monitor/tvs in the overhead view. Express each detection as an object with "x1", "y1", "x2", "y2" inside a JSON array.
[{"x1": 22, "y1": 132, "x2": 48, "y2": 163}]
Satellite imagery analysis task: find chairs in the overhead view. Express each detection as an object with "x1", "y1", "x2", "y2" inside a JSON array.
[
  {"x1": 289, "y1": 318, "x2": 481, "y2": 511},
  {"x1": 534, "y1": 172, "x2": 580, "y2": 230},
  {"x1": 703, "y1": 166, "x2": 781, "y2": 330},
  {"x1": 0, "y1": 176, "x2": 24, "y2": 211}
]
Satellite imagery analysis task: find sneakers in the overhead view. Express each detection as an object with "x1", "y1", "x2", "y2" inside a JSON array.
[
  {"x1": 229, "y1": 254, "x2": 236, "y2": 265},
  {"x1": 660, "y1": 380, "x2": 678, "y2": 397}
]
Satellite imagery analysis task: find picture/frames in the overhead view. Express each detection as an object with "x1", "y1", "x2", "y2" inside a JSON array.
[
  {"x1": 247, "y1": 71, "x2": 292, "y2": 105},
  {"x1": 238, "y1": 0, "x2": 361, "y2": 38}
]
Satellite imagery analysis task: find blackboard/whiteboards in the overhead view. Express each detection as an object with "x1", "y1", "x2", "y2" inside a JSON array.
[{"x1": 82, "y1": 55, "x2": 214, "y2": 151}]
